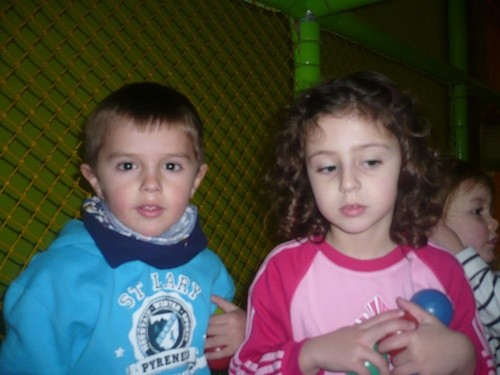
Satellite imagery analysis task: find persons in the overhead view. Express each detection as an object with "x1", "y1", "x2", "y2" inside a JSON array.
[
  {"x1": 230, "y1": 79, "x2": 497, "y2": 375},
  {"x1": 0, "y1": 82, "x2": 247, "y2": 375},
  {"x1": 422, "y1": 159, "x2": 500, "y2": 375}
]
